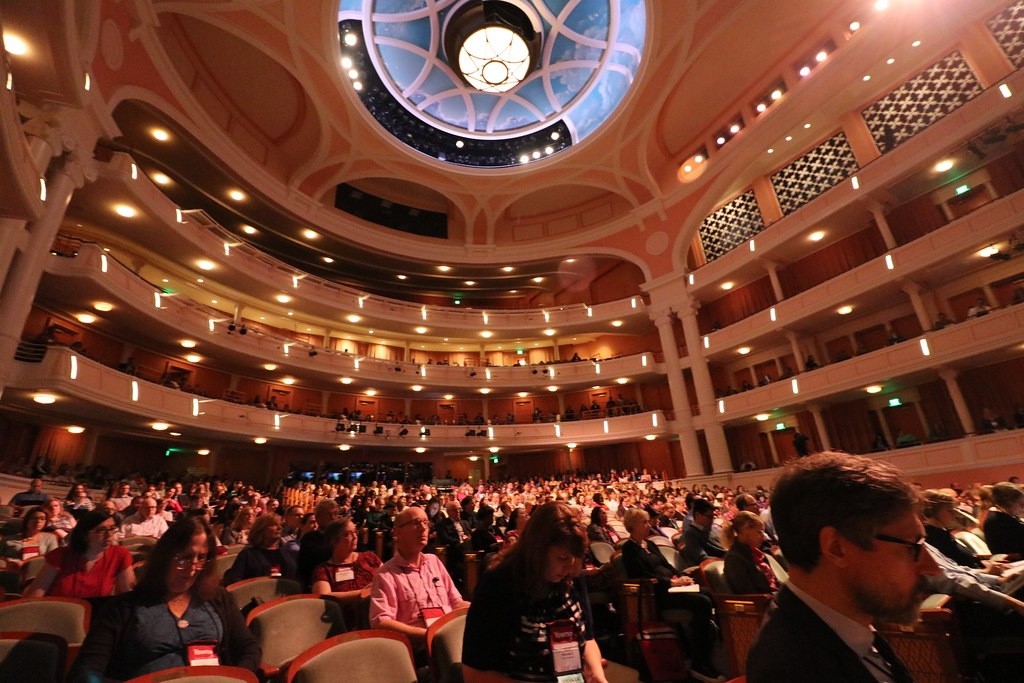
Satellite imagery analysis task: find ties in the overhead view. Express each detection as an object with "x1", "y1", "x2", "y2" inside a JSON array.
[{"x1": 872, "y1": 630, "x2": 913, "y2": 683}]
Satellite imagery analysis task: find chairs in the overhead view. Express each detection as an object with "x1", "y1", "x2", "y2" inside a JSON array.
[
  {"x1": 0, "y1": 494, "x2": 610, "y2": 683},
  {"x1": 281, "y1": 488, "x2": 993, "y2": 682}
]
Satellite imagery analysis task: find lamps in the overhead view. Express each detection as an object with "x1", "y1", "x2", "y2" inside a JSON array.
[
  {"x1": 419, "y1": 429, "x2": 430, "y2": 436},
  {"x1": 440, "y1": 0, "x2": 545, "y2": 93},
  {"x1": 335, "y1": 423, "x2": 366, "y2": 433},
  {"x1": 373, "y1": 426, "x2": 383, "y2": 434},
  {"x1": 399, "y1": 428, "x2": 408, "y2": 436},
  {"x1": 464, "y1": 430, "x2": 487, "y2": 436}
]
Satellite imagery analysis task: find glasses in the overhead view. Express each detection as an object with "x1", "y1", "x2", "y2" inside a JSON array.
[
  {"x1": 703, "y1": 513, "x2": 714, "y2": 519},
  {"x1": 174, "y1": 554, "x2": 210, "y2": 570},
  {"x1": 399, "y1": 518, "x2": 432, "y2": 527},
  {"x1": 88, "y1": 525, "x2": 119, "y2": 535},
  {"x1": 748, "y1": 502, "x2": 758, "y2": 506},
  {"x1": 873, "y1": 532, "x2": 925, "y2": 563}
]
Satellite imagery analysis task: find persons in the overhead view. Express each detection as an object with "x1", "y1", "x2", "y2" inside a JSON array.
[
  {"x1": 982, "y1": 408, "x2": 1010, "y2": 435},
  {"x1": 912, "y1": 476, "x2": 1024, "y2": 669},
  {"x1": 439, "y1": 501, "x2": 472, "y2": 584},
  {"x1": 792, "y1": 433, "x2": 811, "y2": 460},
  {"x1": 297, "y1": 467, "x2": 766, "y2": 557},
  {"x1": 462, "y1": 501, "x2": 607, "y2": 683},
  {"x1": 22, "y1": 510, "x2": 136, "y2": 604},
  {"x1": 745, "y1": 451, "x2": 942, "y2": 683},
  {"x1": 100, "y1": 483, "x2": 342, "y2": 591},
  {"x1": 711, "y1": 286, "x2": 1024, "y2": 399},
  {"x1": 74, "y1": 515, "x2": 267, "y2": 683},
  {"x1": 313, "y1": 519, "x2": 383, "y2": 621},
  {"x1": 40, "y1": 498, "x2": 77, "y2": 544},
  {"x1": 369, "y1": 508, "x2": 471, "y2": 649},
  {"x1": 4, "y1": 508, "x2": 58, "y2": 568},
  {"x1": 623, "y1": 510, "x2": 721, "y2": 676},
  {"x1": 723, "y1": 510, "x2": 781, "y2": 598},
  {"x1": 11, "y1": 479, "x2": 50, "y2": 506},
  {"x1": 33, "y1": 318, "x2": 642, "y2": 426},
  {"x1": 63, "y1": 484, "x2": 92, "y2": 514}
]
